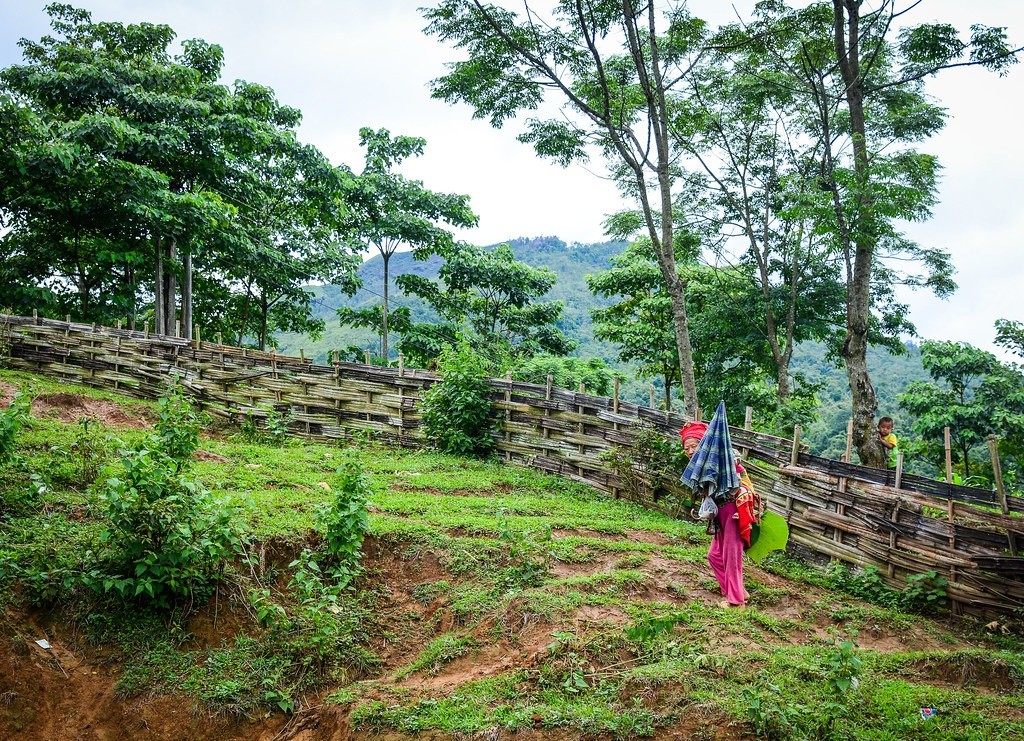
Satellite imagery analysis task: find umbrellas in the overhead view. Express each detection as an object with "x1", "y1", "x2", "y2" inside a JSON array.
[{"x1": 679, "y1": 392, "x2": 741, "y2": 520}]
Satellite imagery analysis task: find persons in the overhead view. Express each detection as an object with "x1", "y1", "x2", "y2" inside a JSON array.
[
  {"x1": 876, "y1": 416, "x2": 901, "y2": 488},
  {"x1": 729, "y1": 447, "x2": 755, "y2": 519},
  {"x1": 679, "y1": 419, "x2": 759, "y2": 611}
]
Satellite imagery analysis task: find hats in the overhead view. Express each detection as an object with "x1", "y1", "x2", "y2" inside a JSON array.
[{"x1": 679, "y1": 421, "x2": 707, "y2": 443}]
